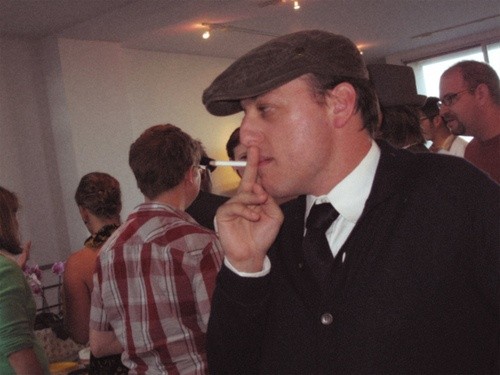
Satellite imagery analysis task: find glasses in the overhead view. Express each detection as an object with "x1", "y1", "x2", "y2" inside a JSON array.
[
  {"x1": 419, "y1": 113, "x2": 436, "y2": 124},
  {"x1": 437, "y1": 88, "x2": 474, "y2": 108}
]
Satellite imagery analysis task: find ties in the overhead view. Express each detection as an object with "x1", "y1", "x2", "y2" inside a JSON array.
[{"x1": 303, "y1": 202, "x2": 340, "y2": 278}]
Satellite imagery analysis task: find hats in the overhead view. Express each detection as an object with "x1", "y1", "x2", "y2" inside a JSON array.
[
  {"x1": 363, "y1": 63, "x2": 428, "y2": 109},
  {"x1": 201, "y1": 28, "x2": 369, "y2": 118}
]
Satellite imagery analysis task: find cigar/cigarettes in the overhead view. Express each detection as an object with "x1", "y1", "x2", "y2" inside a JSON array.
[{"x1": 209, "y1": 160, "x2": 248, "y2": 168}]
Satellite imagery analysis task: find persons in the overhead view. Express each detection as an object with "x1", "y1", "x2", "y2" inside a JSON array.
[
  {"x1": 366, "y1": 63, "x2": 432, "y2": 154},
  {"x1": 0, "y1": 187, "x2": 51, "y2": 375},
  {"x1": 63, "y1": 171, "x2": 129, "y2": 375},
  {"x1": 438, "y1": 60, "x2": 499, "y2": 183},
  {"x1": 418, "y1": 96, "x2": 469, "y2": 158},
  {"x1": 172, "y1": 128, "x2": 300, "y2": 234},
  {"x1": 89, "y1": 122, "x2": 225, "y2": 375},
  {"x1": 202, "y1": 29, "x2": 500, "y2": 375}
]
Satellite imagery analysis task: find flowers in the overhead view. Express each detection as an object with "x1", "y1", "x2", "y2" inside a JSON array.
[
  {"x1": 21, "y1": 264, "x2": 43, "y2": 297},
  {"x1": 50, "y1": 260, "x2": 65, "y2": 277}
]
